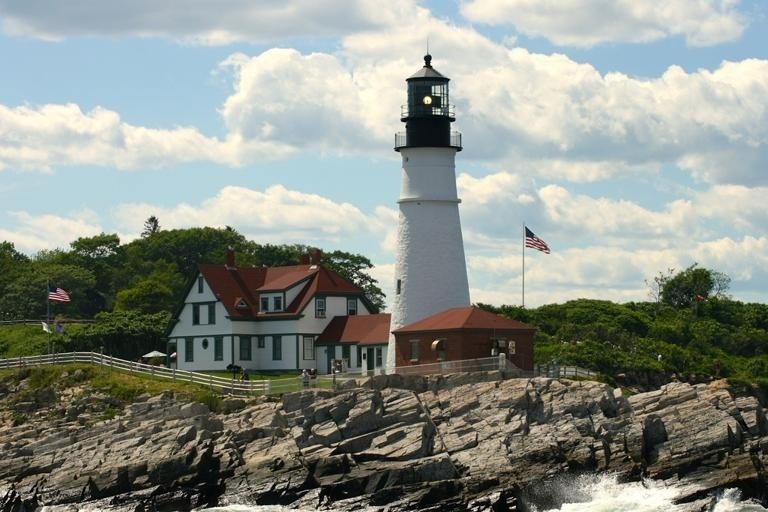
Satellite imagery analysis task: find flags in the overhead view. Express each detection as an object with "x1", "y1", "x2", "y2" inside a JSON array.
[
  {"x1": 41, "y1": 322, "x2": 52, "y2": 334},
  {"x1": 524, "y1": 226, "x2": 550, "y2": 254},
  {"x1": 55, "y1": 322, "x2": 68, "y2": 337},
  {"x1": 47, "y1": 283, "x2": 71, "y2": 305}
]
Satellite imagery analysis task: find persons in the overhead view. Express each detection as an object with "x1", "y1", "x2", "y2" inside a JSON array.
[
  {"x1": 309, "y1": 368, "x2": 317, "y2": 388},
  {"x1": 301, "y1": 369, "x2": 309, "y2": 389},
  {"x1": 239, "y1": 370, "x2": 249, "y2": 395}
]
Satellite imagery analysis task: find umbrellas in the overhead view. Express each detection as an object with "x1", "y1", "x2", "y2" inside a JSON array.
[{"x1": 142, "y1": 351, "x2": 167, "y2": 358}]
